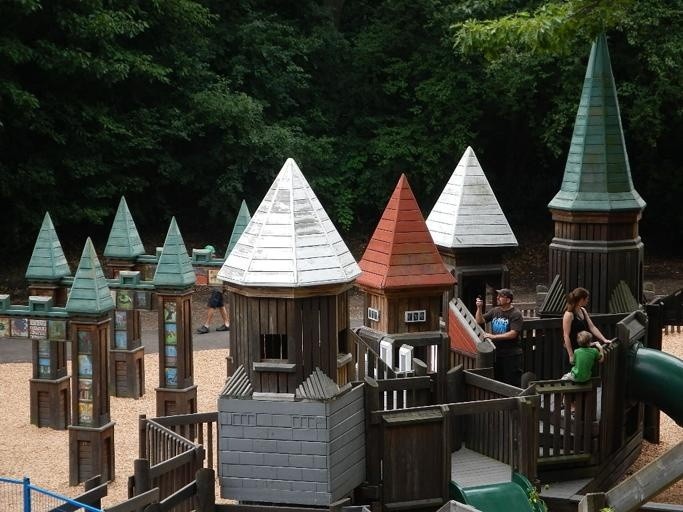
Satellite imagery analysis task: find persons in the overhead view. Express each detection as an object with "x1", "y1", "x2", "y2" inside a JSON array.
[
  {"x1": 560, "y1": 287, "x2": 611, "y2": 419},
  {"x1": 197, "y1": 292, "x2": 231, "y2": 334},
  {"x1": 560, "y1": 331, "x2": 604, "y2": 410},
  {"x1": 476, "y1": 287, "x2": 525, "y2": 389}
]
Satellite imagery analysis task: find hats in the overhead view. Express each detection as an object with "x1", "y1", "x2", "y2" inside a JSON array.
[{"x1": 496, "y1": 289, "x2": 514, "y2": 300}]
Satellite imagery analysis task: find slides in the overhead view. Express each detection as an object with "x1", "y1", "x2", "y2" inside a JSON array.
[
  {"x1": 636, "y1": 345, "x2": 682, "y2": 429},
  {"x1": 449, "y1": 472, "x2": 546, "y2": 512}
]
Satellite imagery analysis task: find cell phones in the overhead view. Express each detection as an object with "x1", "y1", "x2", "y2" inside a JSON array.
[{"x1": 478, "y1": 295, "x2": 482, "y2": 300}]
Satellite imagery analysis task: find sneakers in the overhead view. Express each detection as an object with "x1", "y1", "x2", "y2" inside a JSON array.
[
  {"x1": 197, "y1": 326, "x2": 210, "y2": 333},
  {"x1": 215, "y1": 324, "x2": 229, "y2": 331}
]
